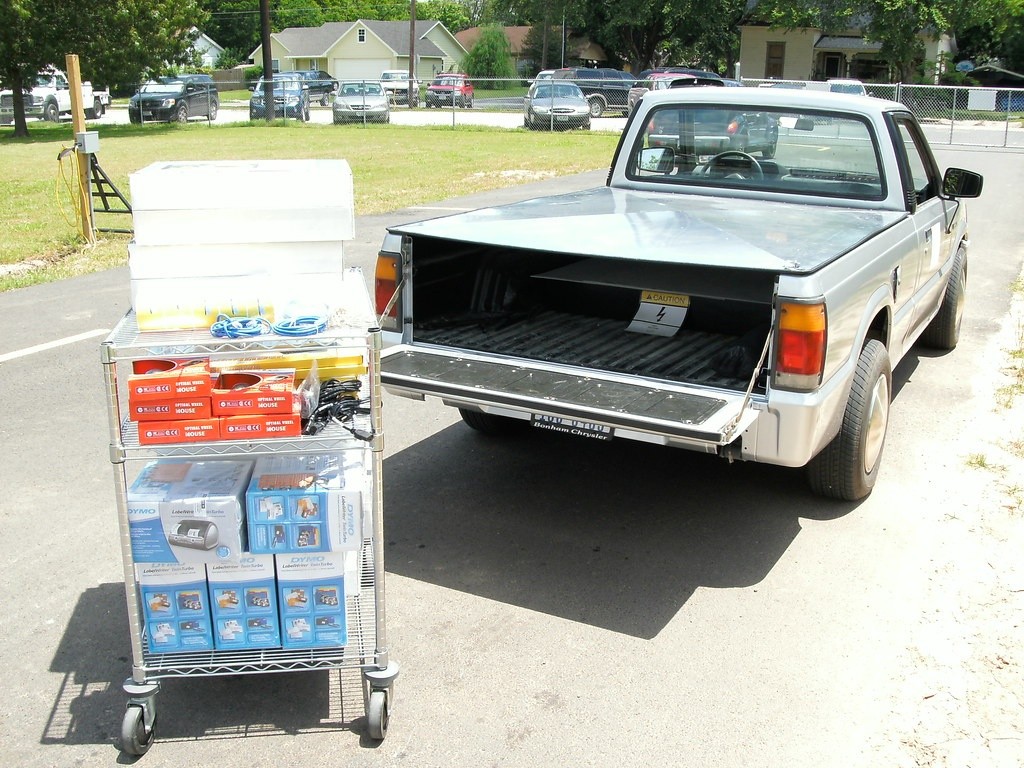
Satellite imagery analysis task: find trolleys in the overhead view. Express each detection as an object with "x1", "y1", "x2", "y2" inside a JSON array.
[{"x1": 99, "y1": 318, "x2": 400, "y2": 755}]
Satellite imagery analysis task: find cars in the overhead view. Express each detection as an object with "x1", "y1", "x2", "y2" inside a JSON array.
[
  {"x1": 248, "y1": 70, "x2": 339, "y2": 123},
  {"x1": 523, "y1": 67, "x2": 745, "y2": 130},
  {"x1": 425, "y1": 73, "x2": 474, "y2": 108},
  {"x1": 128, "y1": 74, "x2": 220, "y2": 124},
  {"x1": 330, "y1": 70, "x2": 423, "y2": 125}
]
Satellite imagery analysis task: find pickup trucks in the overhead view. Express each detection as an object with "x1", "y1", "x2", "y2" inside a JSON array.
[
  {"x1": 374, "y1": 86, "x2": 985, "y2": 503},
  {"x1": 0, "y1": 63, "x2": 110, "y2": 123}
]
